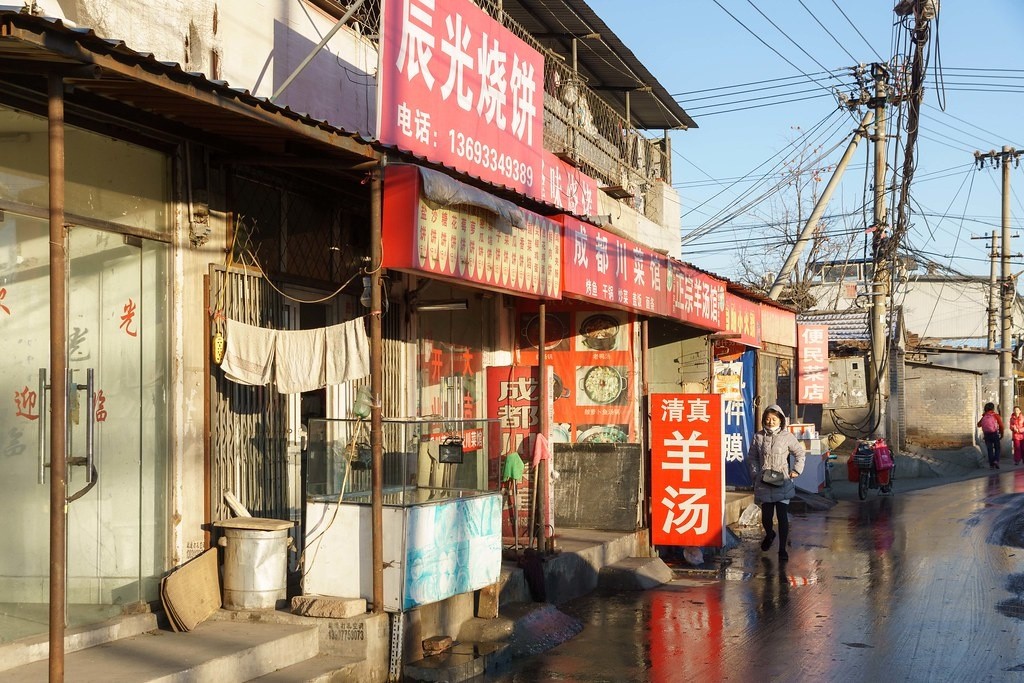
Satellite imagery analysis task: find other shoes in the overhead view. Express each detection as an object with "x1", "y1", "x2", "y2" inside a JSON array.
[
  {"x1": 989, "y1": 465, "x2": 995, "y2": 470},
  {"x1": 778, "y1": 549, "x2": 789, "y2": 563},
  {"x1": 1015, "y1": 461, "x2": 1019, "y2": 465},
  {"x1": 760, "y1": 530, "x2": 776, "y2": 551},
  {"x1": 994, "y1": 461, "x2": 999, "y2": 469}
]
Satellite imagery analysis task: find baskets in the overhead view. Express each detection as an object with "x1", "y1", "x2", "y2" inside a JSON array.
[{"x1": 852, "y1": 452, "x2": 874, "y2": 468}]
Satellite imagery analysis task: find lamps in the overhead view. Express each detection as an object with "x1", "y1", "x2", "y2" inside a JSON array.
[{"x1": 439, "y1": 437, "x2": 464, "y2": 464}]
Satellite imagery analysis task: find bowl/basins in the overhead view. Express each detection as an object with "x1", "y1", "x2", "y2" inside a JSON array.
[
  {"x1": 553, "y1": 425, "x2": 570, "y2": 442},
  {"x1": 576, "y1": 427, "x2": 628, "y2": 442}
]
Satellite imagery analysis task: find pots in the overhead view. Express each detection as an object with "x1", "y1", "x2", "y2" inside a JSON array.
[
  {"x1": 579, "y1": 366, "x2": 628, "y2": 404},
  {"x1": 521, "y1": 314, "x2": 568, "y2": 350},
  {"x1": 579, "y1": 314, "x2": 620, "y2": 351},
  {"x1": 553, "y1": 372, "x2": 570, "y2": 402}
]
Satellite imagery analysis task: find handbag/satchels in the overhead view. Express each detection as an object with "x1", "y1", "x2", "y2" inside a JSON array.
[
  {"x1": 761, "y1": 469, "x2": 785, "y2": 488},
  {"x1": 854, "y1": 441, "x2": 872, "y2": 464},
  {"x1": 872, "y1": 438, "x2": 894, "y2": 471}
]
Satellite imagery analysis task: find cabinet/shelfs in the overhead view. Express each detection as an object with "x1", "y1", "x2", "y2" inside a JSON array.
[
  {"x1": 789, "y1": 435, "x2": 830, "y2": 493},
  {"x1": 304, "y1": 416, "x2": 502, "y2": 613}
]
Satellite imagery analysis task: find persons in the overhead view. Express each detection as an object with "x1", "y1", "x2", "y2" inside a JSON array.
[
  {"x1": 748, "y1": 405, "x2": 805, "y2": 560},
  {"x1": 1010, "y1": 406, "x2": 1024, "y2": 465},
  {"x1": 977, "y1": 402, "x2": 1004, "y2": 470}
]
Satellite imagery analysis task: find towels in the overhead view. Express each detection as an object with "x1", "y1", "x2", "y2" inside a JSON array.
[
  {"x1": 220, "y1": 317, "x2": 277, "y2": 386},
  {"x1": 326, "y1": 316, "x2": 370, "y2": 385},
  {"x1": 275, "y1": 327, "x2": 326, "y2": 395}
]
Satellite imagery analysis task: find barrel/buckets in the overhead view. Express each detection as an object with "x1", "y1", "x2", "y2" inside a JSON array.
[{"x1": 222, "y1": 528, "x2": 288, "y2": 612}]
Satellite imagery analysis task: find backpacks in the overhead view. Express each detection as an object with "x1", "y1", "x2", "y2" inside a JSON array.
[{"x1": 981, "y1": 413, "x2": 997, "y2": 433}]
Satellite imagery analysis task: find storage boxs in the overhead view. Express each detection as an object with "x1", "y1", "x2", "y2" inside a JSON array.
[{"x1": 787, "y1": 423, "x2": 816, "y2": 439}]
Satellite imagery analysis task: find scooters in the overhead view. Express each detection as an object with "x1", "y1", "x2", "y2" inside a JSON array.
[{"x1": 853, "y1": 436, "x2": 896, "y2": 500}]
